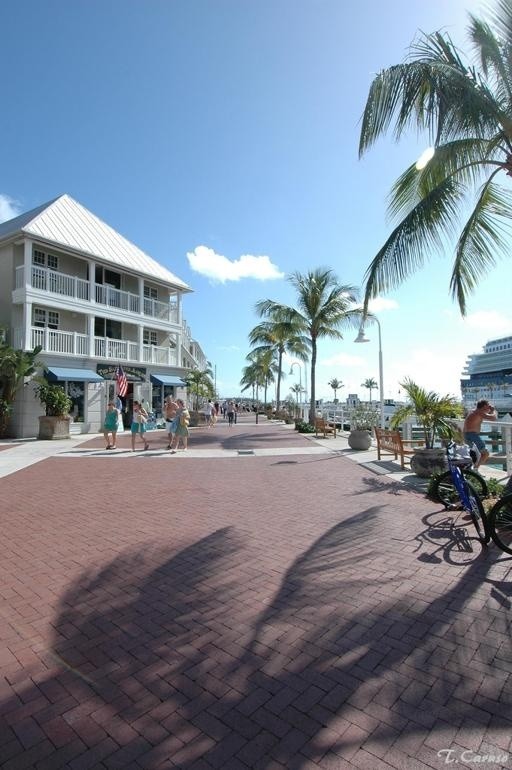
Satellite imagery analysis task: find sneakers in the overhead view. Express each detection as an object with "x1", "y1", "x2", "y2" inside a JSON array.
[
  {"x1": 166, "y1": 443, "x2": 187, "y2": 454},
  {"x1": 106, "y1": 443, "x2": 150, "y2": 452}
]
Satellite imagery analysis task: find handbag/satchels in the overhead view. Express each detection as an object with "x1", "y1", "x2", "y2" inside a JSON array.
[
  {"x1": 138, "y1": 414, "x2": 147, "y2": 423},
  {"x1": 180, "y1": 417, "x2": 189, "y2": 426}
]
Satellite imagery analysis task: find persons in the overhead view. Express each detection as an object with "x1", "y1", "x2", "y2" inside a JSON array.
[
  {"x1": 105, "y1": 402, "x2": 120, "y2": 450},
  {"x1": 161, "y1": 394, "x2": 179, "y2": 449},
  {"x1": 203, "y1": 397, "x2": 263, "y2": 428},
  {"x1": 463, "y1": 400, "x2": 498, "y2": 477},
  {"x1": 130, "y1": 400, "x2": 150, "y2": 453},
  {"x1": 169, "y1": 399, "x2": 190, "y2": 453}
]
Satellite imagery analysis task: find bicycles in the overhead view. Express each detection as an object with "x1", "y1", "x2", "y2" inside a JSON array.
[{"x1": 433, "y1": 417, "x2": 512, "y2": 556}]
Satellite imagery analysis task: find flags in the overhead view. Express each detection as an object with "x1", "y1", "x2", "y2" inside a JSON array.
[{"x1": 116, "y1": 363, "x2": 128, "y2": 398}]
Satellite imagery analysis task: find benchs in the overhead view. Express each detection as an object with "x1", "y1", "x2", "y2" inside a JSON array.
[
  {"x1": 374, "y1": 427, "x2": 426, "y2": 471},
  {"x1": 314, "y1": 418, "x2": 336, "y2": 438}
]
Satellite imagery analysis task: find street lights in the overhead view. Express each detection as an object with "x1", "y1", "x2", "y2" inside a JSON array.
[
  {"x1": 353, "y1": 315, "x2": 386, "y2": 450},
  {"x1": 288, "y1": 362, "x2": 303, "y2": 419}
]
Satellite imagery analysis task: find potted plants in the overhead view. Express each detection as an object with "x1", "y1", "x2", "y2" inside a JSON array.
[
  {"x1": 344, "y1": 406, "x2": 377, "y2": 451},
  {"x1": 33, "y1": 385, "x2": 73, "y2": 439},
  {"x1": 389, "y1": 375, "x2": 464, "y2": 478},
  {"x1": 180, "y1": 368, "x2": 214, "y2": 427}
]
